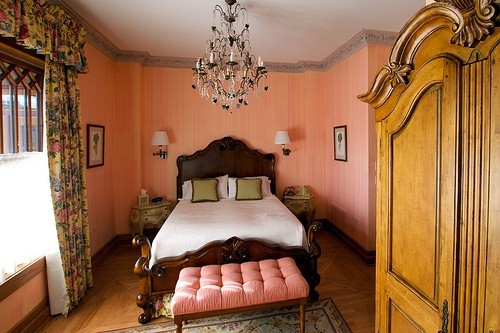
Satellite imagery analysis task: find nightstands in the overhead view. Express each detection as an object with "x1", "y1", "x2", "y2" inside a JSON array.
[
  {"x1": 284, "y1": 194, "x2": 315, "y2": 228},
  {"x1": 131, "y1": 200, "x2": 172, "y2": 235}
]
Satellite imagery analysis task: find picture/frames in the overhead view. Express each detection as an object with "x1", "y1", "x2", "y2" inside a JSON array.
[
  {"x1": 86, "y1": 123, "x2": 105, "y2": 168},
  {"x1": 333, "y1": 125, "x2": 347, "y2": 161}
]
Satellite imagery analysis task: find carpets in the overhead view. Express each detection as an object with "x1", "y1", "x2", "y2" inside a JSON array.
[{"x1": 91, "y1": 297, "x2": 353, "y2": 333}]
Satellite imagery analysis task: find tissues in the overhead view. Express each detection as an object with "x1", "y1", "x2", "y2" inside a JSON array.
[{"x1": 136, "y1": 188, "x2": 149, "y2": 207}]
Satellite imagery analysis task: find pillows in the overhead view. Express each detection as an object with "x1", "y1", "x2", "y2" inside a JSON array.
[{"x1": 181, "y1": 174, "x2": 272, "y2": 203}]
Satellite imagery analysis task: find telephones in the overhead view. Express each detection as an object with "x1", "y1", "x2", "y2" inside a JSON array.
[{"x1": 284, "y1": 186, "x2": 295, "y2": 196}]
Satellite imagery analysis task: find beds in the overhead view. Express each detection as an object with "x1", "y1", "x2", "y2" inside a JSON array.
[{"x1": 132, "y1": 136, "x2": 322, "y2": 324}]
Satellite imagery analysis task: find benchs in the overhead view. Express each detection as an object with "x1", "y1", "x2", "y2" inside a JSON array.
[{"x1": 170, "y1": 257, "x2": 310, "y2": 333}]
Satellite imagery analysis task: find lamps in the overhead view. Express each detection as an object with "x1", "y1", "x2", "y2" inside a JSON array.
[
  {"x1": 150, "y1": 131, "x2": 169, "y2": 159},
  {"x1": 274, "y1": 130, "x2": 291, "y2": 155},
  {"x1": 192, "y1": 0, "x2": 268, "y2": 115}
]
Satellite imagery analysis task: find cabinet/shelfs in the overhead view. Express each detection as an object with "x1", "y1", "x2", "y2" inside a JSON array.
[{"x1": 356, "y1": 0, "x2": 500, "y2": 333}]
